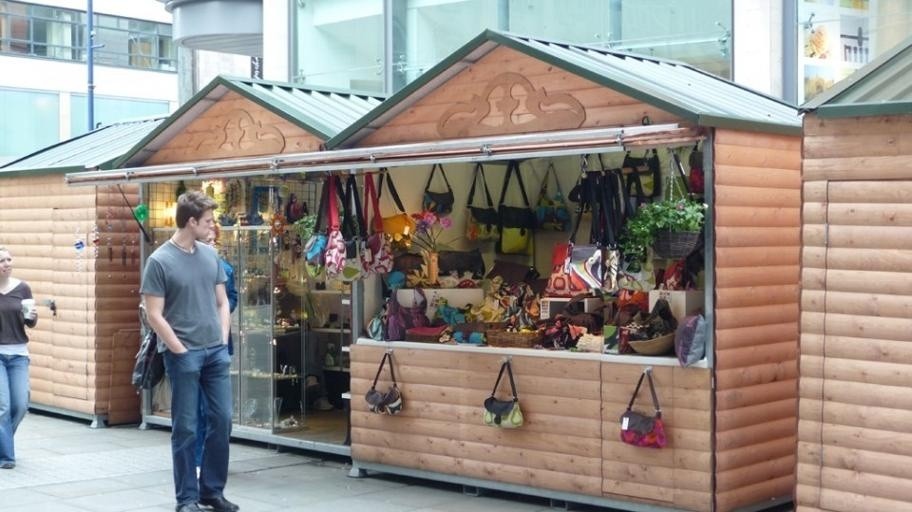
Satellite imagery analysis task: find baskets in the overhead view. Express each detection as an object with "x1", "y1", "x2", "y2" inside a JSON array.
[
  {"x1": 627, "y1": 331, "x2": 674, "y2": 356},
  {"x1": 485, "y1": 329, "x2": 543, "y2": 349},
  {"x1": 455, "y1": 321, "x2": 507, "y2": 340}
]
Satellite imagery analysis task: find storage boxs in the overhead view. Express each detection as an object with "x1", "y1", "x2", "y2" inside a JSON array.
[{"x1": 647, "y1": 288, "x2": 705, "y2": 319}]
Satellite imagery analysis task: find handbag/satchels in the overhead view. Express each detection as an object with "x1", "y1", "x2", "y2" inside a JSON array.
[
  {"x1": 422, "y1": 164, "x2": 454, "y2": 216},
  {"x1": 482, "y1": 257, "x2": 539, "y2": 301},
  {"x1": 618, "y1": 299, "x2": 678, "y2": 355},
  {"x1": 372, "y1": 168, "x2": 416, "y2": 249},
  {"x1": 365, "y1": 352, "x2": 404, "y2": 415},
  {"x1": 540, "y1": 152, "x2": 656, "y2": 299},
  {"x1": 463, "y1": 162, "x2": 500, "y2": 242},
  {"x1": 620, "y1": 367, "x2": 667, "y2": 447},
  {"x1": 621, "y1": 148, "x2": 662, "y2": 197},
  {"x1": 495, "y1": 160, "x2": 536, "y2": 255},
  {"x1": 553, "y1": 288, "x2": 604, "y2": 350},
  {"x1": 482, "y1": 360, "x2": 524, "y2": 429},
  {"x1": 437, "y1": 248, "x2": 486, "y2": 288},
  {"x1": 688, "y1": 142, "x2": 705, "y2": 195},
  {"x1": 533, "y1": 162, "x2": 572, "y2": 232},
  {"x1": 365, "y1": 302, "x2": 390, "y2": 340},
  {"x1": 387, "y1": 288, "x2": 430, "y2": 341},
  {"x1": 404, "y1": 325, "x2": 453, "y2": 343},
  {"x1": 663, "y1": 259, "x2": 698, "y2": 290},
  {"x1": 132, "y1": 329, "x2": 164, "y2": 389},
  {"x1": 302, "y1": 173, "x2": 393, "y2": 282}
]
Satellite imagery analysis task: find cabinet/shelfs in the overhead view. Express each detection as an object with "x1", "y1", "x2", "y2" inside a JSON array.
[
  {"x1": 339, "y1": 298, "x2": 352, "y2": 400},
  {"x1": 153, "y1": 226, "x2": 309, "y2": 436},
  {"x1": 307, "y1": 287, "x2": 352, "y2": 375}
]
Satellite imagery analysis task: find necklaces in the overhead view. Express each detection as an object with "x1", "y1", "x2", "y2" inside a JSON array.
[{"x1": 171, "y1": 237, "x2": 196, "y2": 253}]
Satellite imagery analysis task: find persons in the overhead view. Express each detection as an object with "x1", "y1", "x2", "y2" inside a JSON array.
[
  {"x1": 195, "y1": 219, "x2": 238, "y2": 480},
  {"x1": 0, "y1": 245, "x2": 39, "y2": 468},
  {"x1": 137, "y1": 188, "x2": 241, "y2": 512}
]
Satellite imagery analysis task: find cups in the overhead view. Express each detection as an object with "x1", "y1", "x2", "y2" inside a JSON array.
[{"x1": 20, "y1": 299, "x2": 35, "y2": 320}]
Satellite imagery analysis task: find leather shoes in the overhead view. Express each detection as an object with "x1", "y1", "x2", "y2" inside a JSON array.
[
  {"x1": 178, "y1": 502, "x2": 202, "y2": 512},
  {"x1": 197, "y1": 497, "x2": 239, "y2": 512}
]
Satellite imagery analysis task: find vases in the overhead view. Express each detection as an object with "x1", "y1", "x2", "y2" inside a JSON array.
[{"x1": 427, "y1": 253, "x2": 438, "y2": 284}]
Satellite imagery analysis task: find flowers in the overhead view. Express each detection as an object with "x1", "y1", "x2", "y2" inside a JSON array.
[
  {"x1": 620, "y1": 194, "x2": 709, "y2": 260},
  {"x1": 390, "y1": 209, "x2": 478, "y2": 252}
]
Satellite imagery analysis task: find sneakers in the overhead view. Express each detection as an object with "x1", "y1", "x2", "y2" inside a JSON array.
[
  {"x1": 313, "y1": 399, "x2": 334, "y2": 410},
  {"x1": 306, "y1": 375, "x2": 320, "y2": 392}
]
